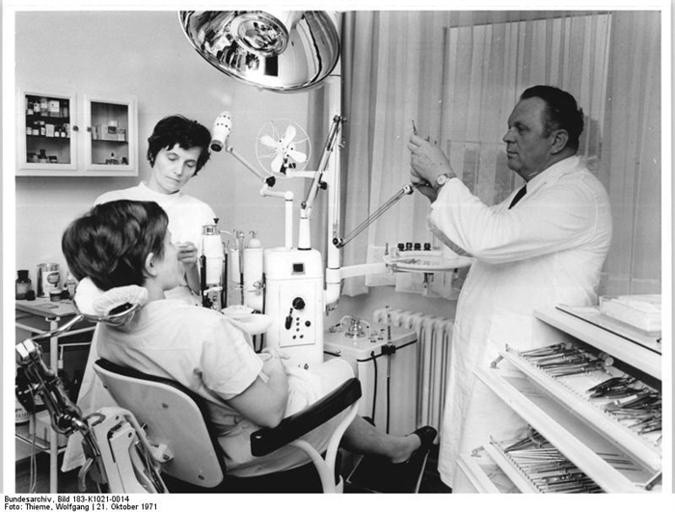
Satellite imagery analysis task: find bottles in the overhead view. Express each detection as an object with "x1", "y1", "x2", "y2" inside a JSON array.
[
  {"x1": 15, "y1": 263, "x2": 76, "y2": 301},
  {"x1": 26, "y1": 122, "x2": 66, "y2": 162}
]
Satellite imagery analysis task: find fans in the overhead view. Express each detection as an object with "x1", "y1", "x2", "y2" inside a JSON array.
[{"x1": 256, "y1": 117, "x2": 311, "y2": 180}]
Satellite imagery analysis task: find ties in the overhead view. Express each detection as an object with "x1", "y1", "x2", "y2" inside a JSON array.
[{"x1": 508, "y1": 185, "x2": 526, "y2": 210}]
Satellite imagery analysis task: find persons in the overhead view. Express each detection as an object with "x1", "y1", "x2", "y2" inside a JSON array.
[
  {"x1": 60, "y1": 116, "x2": 220, "y2": 474},
  {"x1": 62, "y1": 197, "x2": 439, "y2": 479},
  {"x1": 406, "y1": 85, "x2": 614, "y2": 494}
]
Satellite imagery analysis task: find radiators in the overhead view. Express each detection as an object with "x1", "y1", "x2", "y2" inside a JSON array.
[{"x1": 373, "y1": 306, "x2": 452, "y2": 444}]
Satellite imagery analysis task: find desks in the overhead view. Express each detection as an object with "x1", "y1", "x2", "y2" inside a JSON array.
[{"x1": 16, "y1": 298, "x2": 93, "y2": 494}]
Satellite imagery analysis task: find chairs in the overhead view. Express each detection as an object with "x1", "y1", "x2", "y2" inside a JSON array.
[{"x1": 16, "y1": 277, "x2": 362, "y2": 493}]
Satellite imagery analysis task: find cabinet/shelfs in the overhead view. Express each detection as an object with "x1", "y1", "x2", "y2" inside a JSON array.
[
  {"x1": 452, "y1": 305, "x2": 663, "y2": 493},
  {"x1": 16, "y1": 87, "x2": 140, "y2": 179}
]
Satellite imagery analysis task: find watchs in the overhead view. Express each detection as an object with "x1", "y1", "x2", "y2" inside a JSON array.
[{"x1": 433, "y1": 170, "x2": 459, "y2": 192}]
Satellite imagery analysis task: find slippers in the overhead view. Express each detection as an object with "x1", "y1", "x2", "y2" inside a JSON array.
[{"x1": 391, "y1": 426, "x2": 436, "y2": 467}]
